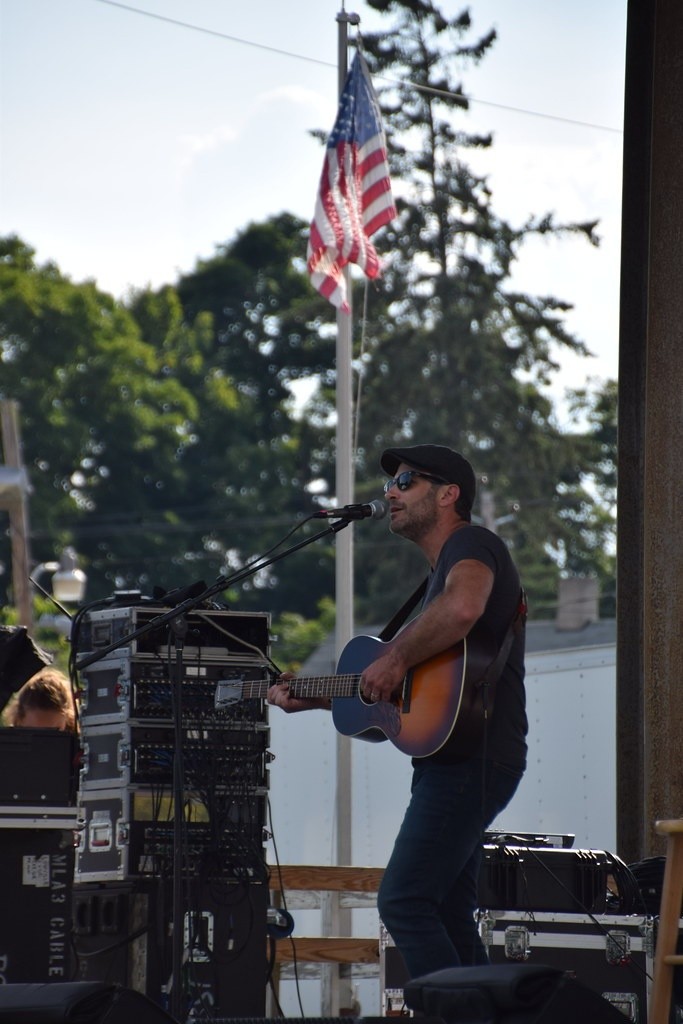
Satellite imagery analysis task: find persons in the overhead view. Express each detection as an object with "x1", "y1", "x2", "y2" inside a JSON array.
[
  {"x1": 266, "y1": 445, "x2": 530, "y2": 980},
  {"x1": 2, "y1": 666, "x2": 81, "y2": 731}
]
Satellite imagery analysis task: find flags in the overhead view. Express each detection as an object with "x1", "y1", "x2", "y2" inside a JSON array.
[{"x1": 307, "y1": 26, "x2": 398, "y2": 315}]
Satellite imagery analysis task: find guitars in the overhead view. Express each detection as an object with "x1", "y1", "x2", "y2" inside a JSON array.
[{"x1": 216, "y1": 612, "x2": 475, "y2": 758}]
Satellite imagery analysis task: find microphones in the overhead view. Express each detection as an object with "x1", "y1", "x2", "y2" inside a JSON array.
[{"x1": 313, "y1": 499, "x2": 387, "y2": 521}]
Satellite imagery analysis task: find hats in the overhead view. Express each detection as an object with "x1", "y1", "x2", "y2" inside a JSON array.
[{"x1": 381, "y1": 443, "x2": 476, "y2": 507}]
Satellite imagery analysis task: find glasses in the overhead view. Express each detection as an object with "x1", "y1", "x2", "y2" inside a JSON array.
[{"x1": 385, "y1": 471, "x2": 449, "y2": 490}]
